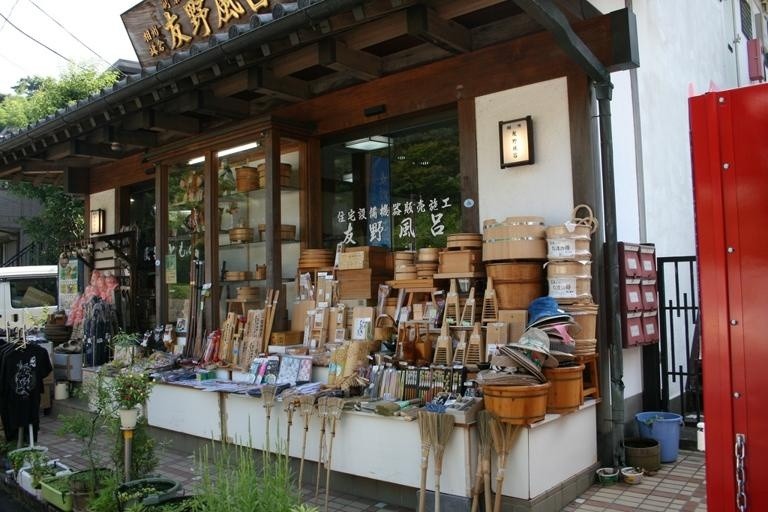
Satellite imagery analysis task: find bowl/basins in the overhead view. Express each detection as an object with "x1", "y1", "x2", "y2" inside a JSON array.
[
  {"x1": 446, "y1": 232, "x2": 481, "y2": 249},
  {"x1": 258, "y1": 223, "x2": 296, "y2": 240},
  {"x1": 482, "y1": 381, "x2": 551, "y2": 424},
  {"x1": 484, "y1": 261, "x2": 544, "y2": 281},
  {"x1": 228, "y1": 228, "x2": 256, "y2": 242},
  {"x1": 299, "y1": 249, "x2": 331, "y2": 267},
  {"x1": 489, "y1": 281, "x2": 542, "y2": 309},
  {"x1": 395, "y1": 247, "x2": 440, "y2": 280},
  {"x1": 483, "y1": 218, "x2": 548, "y2": 259},
  {"x1": 542, "y1": 363, "x2": 585, "y2": 414}
]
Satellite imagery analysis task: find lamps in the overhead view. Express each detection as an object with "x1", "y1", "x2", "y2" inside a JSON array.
[
  {"x1": 89, "y1": 208, "x2": 106, "y2": 236},
  {"x1": 498, "y1": 116, "x2": 534, "y2": 169}
]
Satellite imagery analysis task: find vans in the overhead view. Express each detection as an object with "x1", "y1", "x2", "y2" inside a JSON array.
[{"x1": 0, "y1": 265, "x2": 59, "y2": 345}]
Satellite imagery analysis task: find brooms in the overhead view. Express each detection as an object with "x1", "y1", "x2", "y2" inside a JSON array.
[{"x1": 260, "y1": 384, "x2": 522, "y2": 512}]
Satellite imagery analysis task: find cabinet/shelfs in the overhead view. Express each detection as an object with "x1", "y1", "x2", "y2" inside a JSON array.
[{"x1": 142, "y1": 116, "x2": 322, "y2": 337}]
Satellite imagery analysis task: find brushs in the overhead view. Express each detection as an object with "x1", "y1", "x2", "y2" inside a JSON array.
[
  {"x1": 375, "y1": 403, "x2": 423, "y2": 416},
  {"x1": 378, "y1": 397, "x2": 422, "y2": 411}
]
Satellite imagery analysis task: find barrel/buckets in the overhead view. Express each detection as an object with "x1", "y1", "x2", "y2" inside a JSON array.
[
  {"x1": 235, "y1": 166, "x2": 259, "y2": 192},
  {"x1": 258, "y1": 163, "x2": 291, "y2": 189},
  {"x1": 632, "y1": 412, "x2": 685, "y2": 462},
  {"x1": 626, "y1": 438, "x2": 661, "y2": 470},
  {"x1": 543, "y1": 225, "x2": 592, "y2": 263},
  {"x1": 547, "y1": 260, "x2": 591, "y2": 303},
  {"x1": 558, "y1": 303, "x2": 598, "y2": 356}
]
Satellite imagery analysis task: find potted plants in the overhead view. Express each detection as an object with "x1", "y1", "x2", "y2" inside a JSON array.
[{"x1": 7, "y1": 326, "x2": 203, "y2": 511}]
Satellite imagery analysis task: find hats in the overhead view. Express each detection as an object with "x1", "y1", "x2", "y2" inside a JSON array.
[{"x1": 477, "y1": 297, "x2": 576, "y2": 386}]
